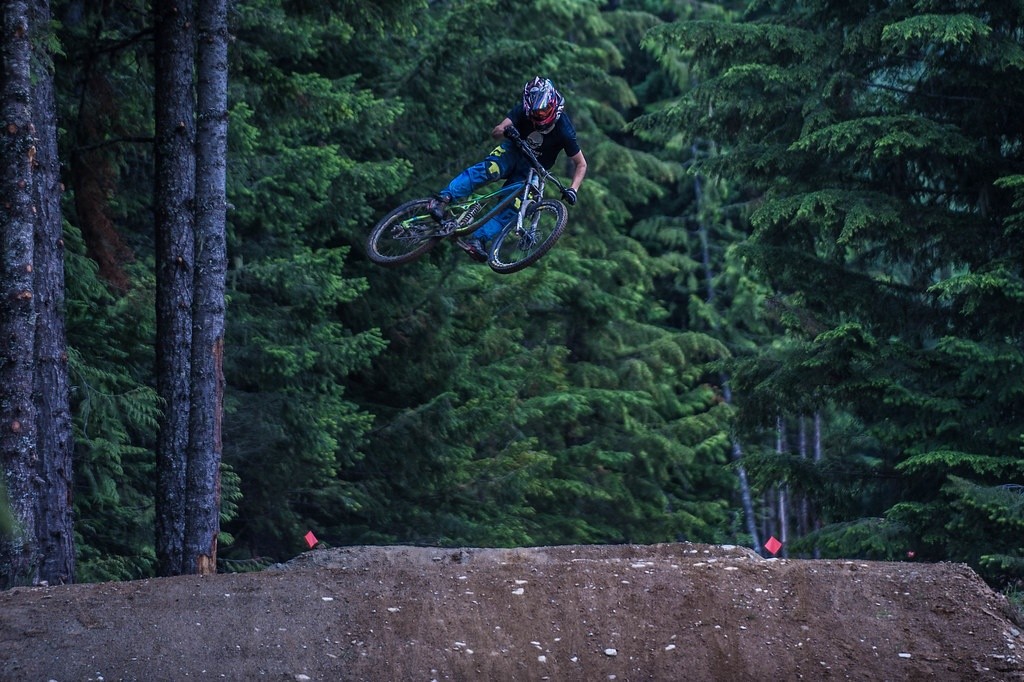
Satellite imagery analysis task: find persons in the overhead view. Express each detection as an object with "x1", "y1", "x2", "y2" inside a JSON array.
[{"x1": 426, "y1": 76, "x2": 587, "y2": 262}]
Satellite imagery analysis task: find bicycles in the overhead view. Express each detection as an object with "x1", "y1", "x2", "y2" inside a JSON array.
[{"x1": 368, "y1": 124, "x2": 575, "y2": 275}]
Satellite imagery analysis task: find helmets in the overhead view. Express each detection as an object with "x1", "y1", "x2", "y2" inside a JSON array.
[{"x1": 523, "y1": 75, "x2": 564, "y2": 136}]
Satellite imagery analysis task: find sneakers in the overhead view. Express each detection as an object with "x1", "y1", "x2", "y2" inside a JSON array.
[
  {"x1": 427, "y1": 194, "x2": 450, "y2": 220},
  {"x1": 456, "y1": 236, "x2": 489, "y2": 263}
]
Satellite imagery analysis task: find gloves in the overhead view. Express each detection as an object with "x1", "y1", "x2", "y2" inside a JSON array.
[{"x1": 565, "y1": 188, "x2": 577, "y2": 205}]
